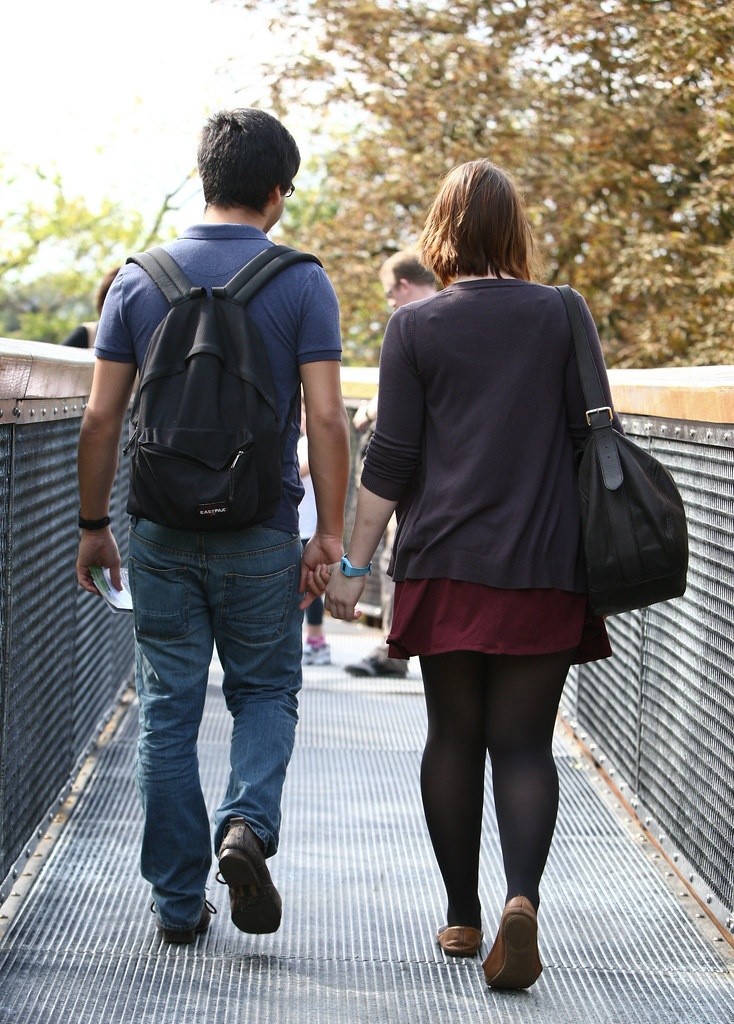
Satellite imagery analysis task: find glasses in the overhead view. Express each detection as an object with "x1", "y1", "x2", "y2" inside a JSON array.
[{"x1": 285, "y1": 182, "x2": 295, "y2": 197}]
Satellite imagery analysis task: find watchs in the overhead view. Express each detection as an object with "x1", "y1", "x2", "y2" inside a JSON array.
[{"x1": 340, "y1": 553, "x2": 373, "y2": 577}]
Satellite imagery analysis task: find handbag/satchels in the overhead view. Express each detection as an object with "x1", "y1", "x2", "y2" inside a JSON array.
[{"x1": 556, "y1": 282, "x2": 689, "y2": 608}]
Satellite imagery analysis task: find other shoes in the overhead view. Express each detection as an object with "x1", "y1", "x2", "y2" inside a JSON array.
[
  {"x1": 344, "y1": 643, "x2": 407, "y2": 678},
  {"x1": 436, "y1": 922, "x2": 482, "y2": 955},
  {"x1": 482, "y1": 896, "x2": 543, "y2": 990}
]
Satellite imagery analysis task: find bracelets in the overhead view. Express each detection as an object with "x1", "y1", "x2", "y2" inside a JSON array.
[
  {"x1": 365, "y1": 403, "x2": 372, "y2": 423},
  {"x1": 78, "y1": 512, "x2": 112, "y2": 530}
]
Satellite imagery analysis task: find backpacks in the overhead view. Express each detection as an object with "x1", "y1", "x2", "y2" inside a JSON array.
[{"x1": 125, "y1": 243, "x2": 324, "y2": 531}]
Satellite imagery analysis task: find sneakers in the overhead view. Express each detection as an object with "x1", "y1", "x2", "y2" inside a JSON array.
[
  {"x1": 215, "y1": 817, "x2": 282, "y2": 933},
  {"x1": 301, "y1": 641, "x2": 332, "y2": 666},
  {"x1": 151, "y1": 888, "x2": 218, "y2": 942}
]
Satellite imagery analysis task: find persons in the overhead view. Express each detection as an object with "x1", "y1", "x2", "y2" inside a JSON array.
[
  {"x1": 294, "y1": 397, "x2": 334, "y2": 666},
  {"x1": 62, "y1": 268, "x2": 121, "y2": 349},
  {"x1": 343, "y1": 252, "x2": 437, "y2": 677},
  {"x1": 323, "y1": 159, "x2": 621, "y2": 991},
  {"x1": 76, "y1": 107, "x2": 350, "y2": 945}
]
